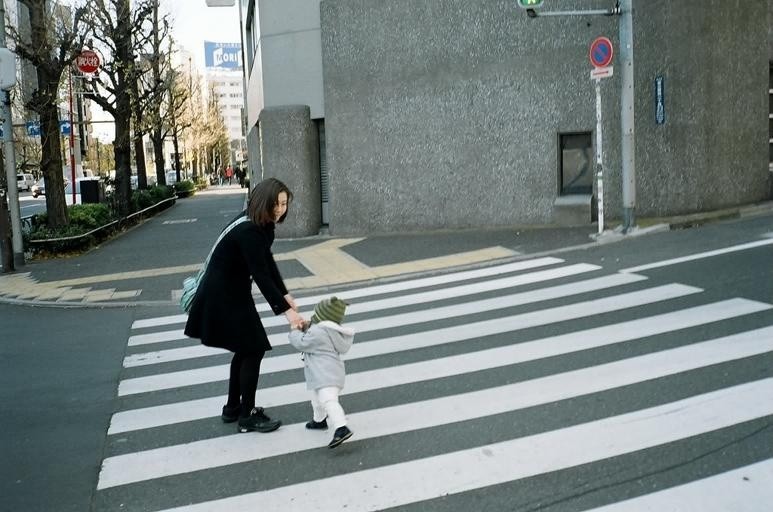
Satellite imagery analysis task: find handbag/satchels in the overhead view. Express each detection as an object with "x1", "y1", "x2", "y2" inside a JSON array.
[{"x1": 178, "y1": 269, "x2": 206, "y2": 315}]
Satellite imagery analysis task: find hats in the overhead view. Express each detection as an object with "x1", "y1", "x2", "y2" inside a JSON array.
[{"x1": 310, "y1": 296, "x2": 349, "y2": 326}]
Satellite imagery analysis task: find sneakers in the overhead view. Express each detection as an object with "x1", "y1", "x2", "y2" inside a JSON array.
[
  {"x1": 222, "y1": 405, "x2": 265, "y2": 424},
  {"x1": 305, "y1": 417, "x2": 328, "y2": 430},
  {"x1": 237, "y1": 407, "x2": 281, "y2": 433},
  {"x1": 327, "y1": 425, "x2": 353, "y2": 449}
]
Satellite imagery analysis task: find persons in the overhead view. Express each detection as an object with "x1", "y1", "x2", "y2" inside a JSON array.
[
  {"x1": 209, "y1": 164, "x2": 246, "y2": 188},
  {"x1": 288, "y1": 296, "x2": 355, "y2": 449},
  {"x1": 183, "y1": 177, "x2": 305, "y2": 433}
]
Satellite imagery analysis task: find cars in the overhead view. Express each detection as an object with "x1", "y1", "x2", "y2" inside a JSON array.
[{"x1": 11, "y1": 168, "x2": 190, "y2": 211}]
